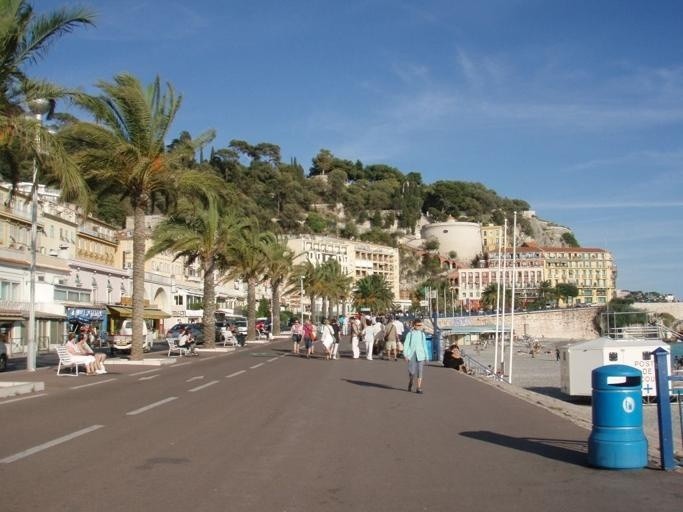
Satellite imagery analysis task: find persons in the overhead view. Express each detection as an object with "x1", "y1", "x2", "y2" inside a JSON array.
[
  {"x1": 74, "y1": 334, "x2": 107, "y2": 375},
  {"x1": 555, "y1": 346, "x2": 560, "y2": 361},
  {"x1": 65, "y1": 333, "x2": 98, "y2": 376},
  {"x1": 402, "y1": 318, "x2": 430, "y2": 394},
  {"x1": 175, "y1": 310, "x2": 403, "y2": 360},
  {"x1": 442, "y1": 344, "x2": 474, "y2": 375}
]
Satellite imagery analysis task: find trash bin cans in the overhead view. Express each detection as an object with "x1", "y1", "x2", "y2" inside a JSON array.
[
  {"x1": 426, "y1": 334, "x2": 433, "y2": 360},
  {"x1": 587, "y1": 365, "x2": 649, "y2": 469}
]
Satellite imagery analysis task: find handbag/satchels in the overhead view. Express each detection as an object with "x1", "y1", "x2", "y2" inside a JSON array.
[
  {"x1": 331, "y1": 334, "x2": 336, "y2": 343},
  {"x1": 397, "y1": 341, "x2": 403, "y2": 351},
  {"x1": 385, "y1": 335, "x2": 388, "y2": 341},
  {"x1": 297, "y1": 335, "x2": 301, "y2": 340}
]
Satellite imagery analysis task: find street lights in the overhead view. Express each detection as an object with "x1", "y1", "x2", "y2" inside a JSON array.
[
  {"x1": 22, "y1": 87, "x2": 63, "y2": 372},
  {"x1": 300, "y1": 275, "x2": 306, "y2": 328},
  {"x1": 420, "y1": 280, "x2": 481, "y2": 320}
]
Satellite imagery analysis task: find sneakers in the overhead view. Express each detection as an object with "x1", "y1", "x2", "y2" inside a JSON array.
[
  {"x1": 409, "y1": 382, "x2": 412, "y2": 391},
  {"x1": 85, "y1": 362, "x2": 107, "y2": 376},
  {"x1": 416, "y1": 388, "x2": 423, "y2": 394}
]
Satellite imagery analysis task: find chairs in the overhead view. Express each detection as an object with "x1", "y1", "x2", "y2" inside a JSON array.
[
  {"x1": 165, "y1": 337, "x2": 186, "y2": 356},
  {"x1": 53, "y1": 341, "x2": 102, "y2": 376},
  {"x1": 220, "y1": 330, "x2": 237, "y2": 347}
]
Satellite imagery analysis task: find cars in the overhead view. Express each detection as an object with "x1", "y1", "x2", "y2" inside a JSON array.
[
  {"x1": 623, "y1": 290, "x2": 675, "y2": 303},
  {"x1": 477, "y1": 301, "x2": 600, "y2": 316},
  {"x1": 114, "y1": 317, "x2": 270, "y2": 353},
  {"x1": 0, "y1": 340, "x2": 9, "y2": 373}
]
